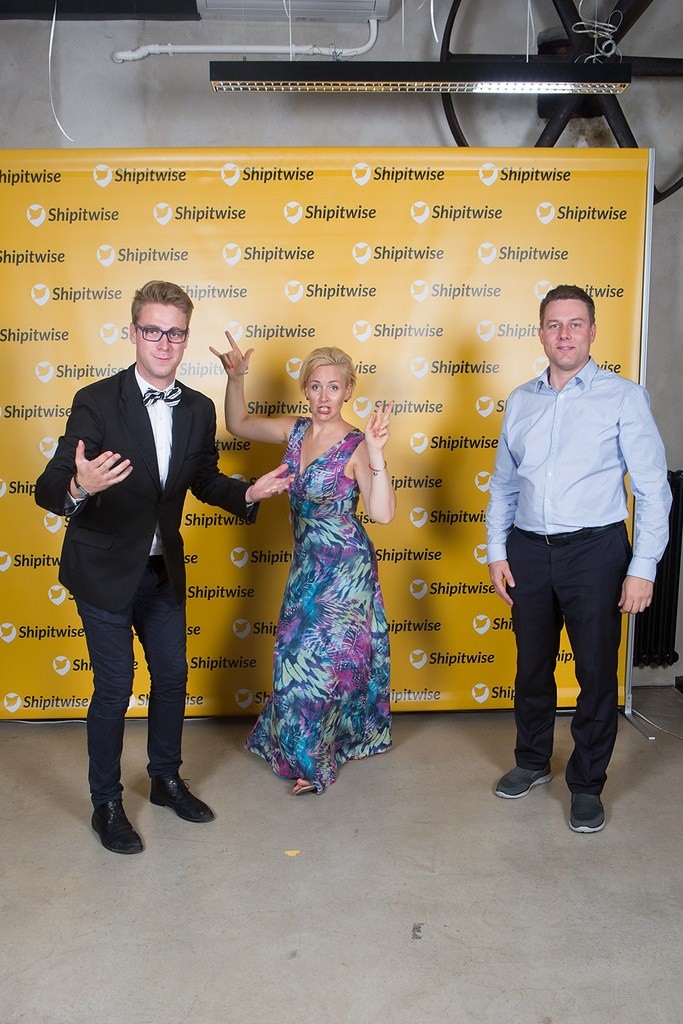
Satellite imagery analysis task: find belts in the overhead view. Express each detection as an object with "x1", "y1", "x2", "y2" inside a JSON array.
[{"x1": 513, "y1": 520, "x2": 624, "y2": 546}]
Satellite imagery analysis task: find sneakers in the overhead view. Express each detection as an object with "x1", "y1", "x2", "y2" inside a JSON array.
[
  {"x1": 569, "y1": 792, "x2": 605, "y2": 833},
  {"x1": 495, "y1": 760, "x2": 553, "y2": 799}
]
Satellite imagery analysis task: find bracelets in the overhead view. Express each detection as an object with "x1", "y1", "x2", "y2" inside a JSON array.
[
  {"x1": 73, "y1": 474, "x2": 90, "y2": 498},
  {"x1": 369, "y1": 460, "x2": 387, "y2": 476}
]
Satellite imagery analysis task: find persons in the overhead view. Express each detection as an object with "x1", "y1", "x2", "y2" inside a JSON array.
[
  {"x1": 486, "y1": 284, "x2": 672, "y2": 834},
  {"x1": 209, "y1": 330, "x2": 396, "y2": 796},
  {"x1": 33, "y1": 280, "x2": 296, "y2": 854}
]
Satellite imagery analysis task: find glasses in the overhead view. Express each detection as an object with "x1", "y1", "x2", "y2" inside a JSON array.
[{"x1": 134, "y1": 323, "x2": 188, "y2": 344}]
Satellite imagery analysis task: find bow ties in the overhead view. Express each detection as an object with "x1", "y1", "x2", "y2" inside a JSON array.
[{"x1": 143, "y1": 387, "x2": 182, "y2": 407}]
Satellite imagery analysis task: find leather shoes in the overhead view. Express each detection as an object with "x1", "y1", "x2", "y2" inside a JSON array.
[
  {"x1": 150, "y1": 771, "x2": 214, "y2": 822},
  {"x1": 92, "y1": 800, "x2": 143, "y2": 854}
]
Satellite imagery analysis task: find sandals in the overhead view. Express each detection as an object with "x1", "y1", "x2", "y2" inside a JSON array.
[{"x1": 291, "y1": 778, "x2": 317, "y2": 795}]
